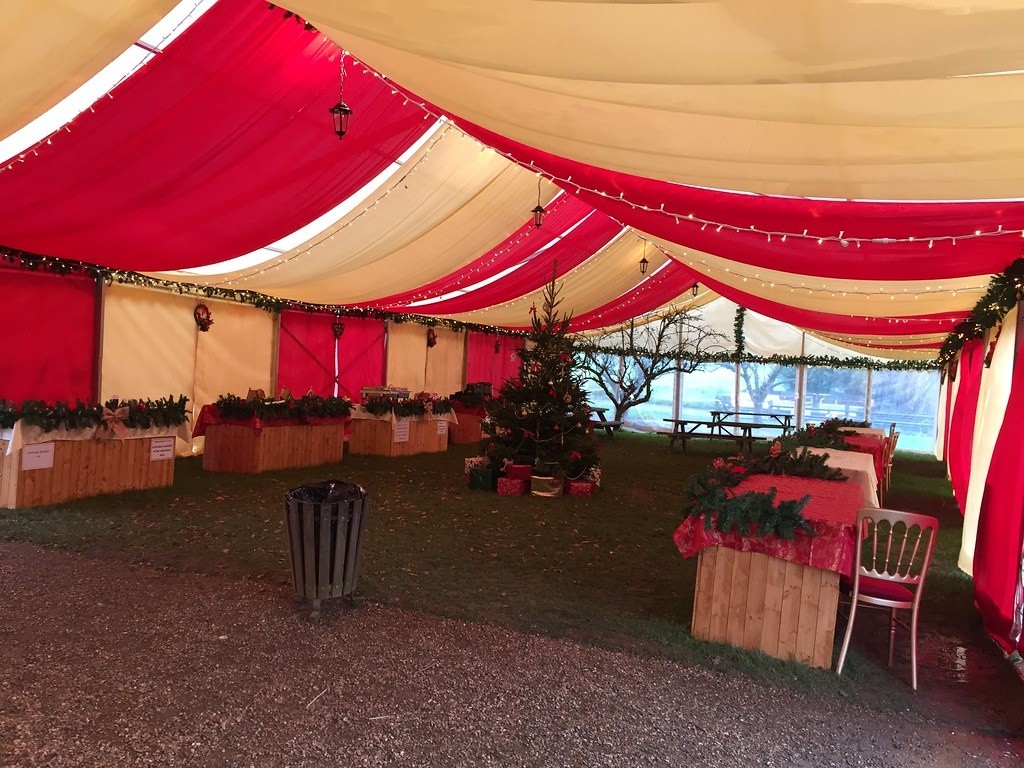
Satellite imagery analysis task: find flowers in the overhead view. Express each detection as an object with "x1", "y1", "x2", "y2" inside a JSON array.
[
  {"x1": 710, "y1": 455, "x2": 724, "y2": 469},
  {"x1": 983, "y1": 325, "x2": 1002, "y2": 370},
  {"x1": 766, "y1": 442, "x2": 783, "y2": 458},
  {"x1": 195, "y1": 303, "x2": 213, "y2": 332}
]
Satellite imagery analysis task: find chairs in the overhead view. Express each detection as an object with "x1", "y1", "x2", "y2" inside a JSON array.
[
  {"x1": 882, "y1": 425, "x2": 899, "y2": 493},
  {"x1": 837, "y1": 507, "x2": 940, "y2": 690}
]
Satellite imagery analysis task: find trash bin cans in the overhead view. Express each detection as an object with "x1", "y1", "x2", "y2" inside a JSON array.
[{"x1": 279, "y1": 478, "x2": 371, "y2": 610}]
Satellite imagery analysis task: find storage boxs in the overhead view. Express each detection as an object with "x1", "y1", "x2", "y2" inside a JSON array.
[
  {"x1": 497, "y1": 477, "x2": 528, "y2": 496},
  {"x1": 506, "y1": 464, "x2": 532, "y2": 480},
  {"x1": 464, "y1": 455, "x2": 490, "y2": 483},
  {"x1": 530, "y1": 476, "x2": 566, "y2": 497},
  {"x1": 469, "y1": 467, "x2": 503, "y2": 492},
  {"x1": 567, "y1": 481, "x2": 591, "y2": 497}
]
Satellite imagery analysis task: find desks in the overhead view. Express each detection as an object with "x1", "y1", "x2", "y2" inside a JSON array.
[
  {"x1": 0, "y1": 415, "x2": 192, "y2": 510},
  {"x1": 586, "y1": 407, "x2": 615, "y2": 437},
  {"x1": 349, "y1": 404, "x2": 459, "y2": 458},
  {"x1": 451, "y1": 401, "x2": 509, "y2": 443},
  {"x1": 672, "y1": 426, "x2": 884, "y2": 670},
  {"x1": 192, "y1": 404, "x2": 352, "y2": 474}
]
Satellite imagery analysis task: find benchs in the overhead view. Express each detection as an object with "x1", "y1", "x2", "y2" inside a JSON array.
[{"x1": 593, "y1": 421, "x2": 624, "y2": 428}]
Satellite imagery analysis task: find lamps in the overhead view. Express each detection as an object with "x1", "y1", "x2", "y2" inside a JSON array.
[
  {"x1": 691, "y1": 281, "x2": 699, "y2": 297},
  {"x1": 531, "y1": 179, "x2": 546, "y2": 226},
  {"x1": 638, "y1": 240, "x2": 650, "y2": 275},
  {"x1": 330, "y1": 54, "x2": 354, "y2": 140}
]
